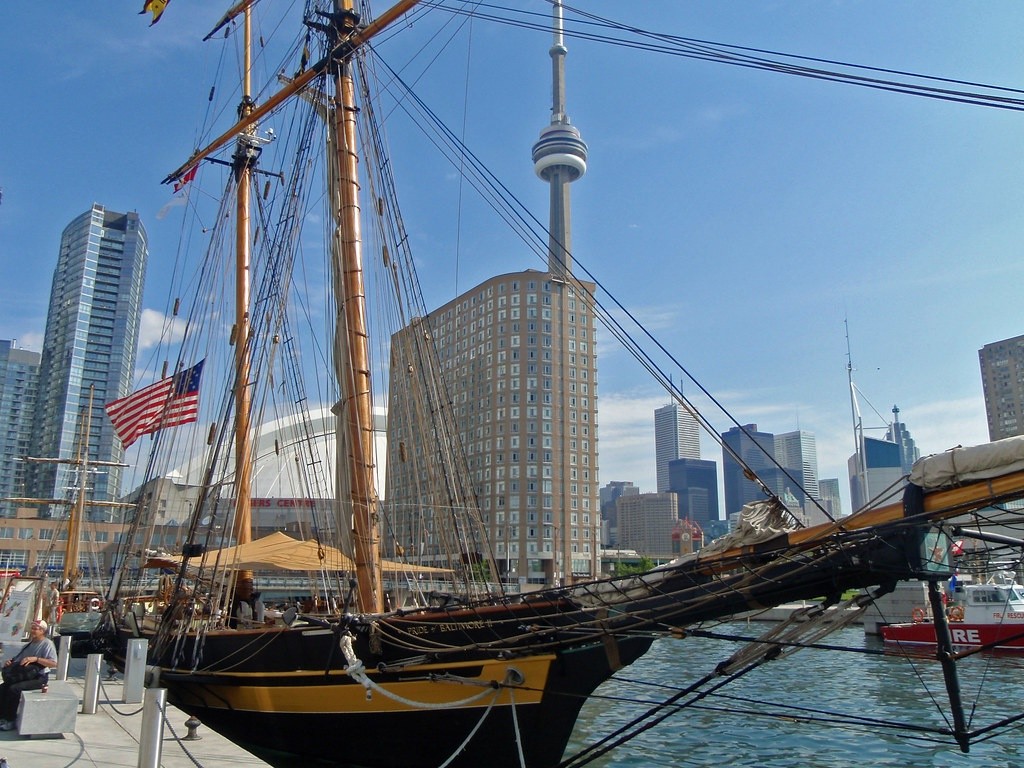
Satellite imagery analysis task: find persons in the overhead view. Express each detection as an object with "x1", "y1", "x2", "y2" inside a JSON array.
[
  {"x1": 0, "y1": 619, "x2": 58, "y2": 731},
  {"x1": 48, "y1": 581, "x2": 59, "y2": 624}
]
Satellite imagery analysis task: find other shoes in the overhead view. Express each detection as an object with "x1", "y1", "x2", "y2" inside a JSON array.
[{"x1": 0, "y1": 719, "x2": 14, "y2": 731}]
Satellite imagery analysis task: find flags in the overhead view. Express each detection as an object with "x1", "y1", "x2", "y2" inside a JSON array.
[{"x1": 104, "y1": 358, "x2": 205, "y2": 452}]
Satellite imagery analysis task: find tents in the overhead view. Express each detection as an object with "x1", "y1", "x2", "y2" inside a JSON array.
[{"x1": 145, "y1": 531, "x2": 456, "y2": 572}]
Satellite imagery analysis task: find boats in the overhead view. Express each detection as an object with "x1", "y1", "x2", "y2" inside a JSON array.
[{"x1": 880, "y1": 517, "x2": 1024, "y2": 651}]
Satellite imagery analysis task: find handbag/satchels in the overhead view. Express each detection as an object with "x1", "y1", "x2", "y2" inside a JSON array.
[{"x1": 2, "y1": 661, "x2": 40, "y2": 686}]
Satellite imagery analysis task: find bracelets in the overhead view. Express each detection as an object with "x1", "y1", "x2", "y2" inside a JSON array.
[{"x1": 37, "y1": 657, "x2": 39, "y2": 663}]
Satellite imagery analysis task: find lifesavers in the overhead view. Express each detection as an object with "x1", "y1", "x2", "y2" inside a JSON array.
[
  {"x1": 912, "y1": 608, "x2": 923, "y2": 621},
  {"x1": 952, "y1": 607, "x2": 963, "y2": 619},
  {"x1": 90, "y1": 599, "x2": 100, "y2": 609}
]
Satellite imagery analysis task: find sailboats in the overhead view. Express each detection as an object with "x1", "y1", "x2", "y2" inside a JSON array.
[{"x1": 0, "y1": 0, "x2": 1023, "y2": 767}]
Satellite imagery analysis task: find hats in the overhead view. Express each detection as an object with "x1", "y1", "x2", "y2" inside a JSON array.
[{"x1": 31, "y1": 620, "x2": 48, "y2": 632}]
